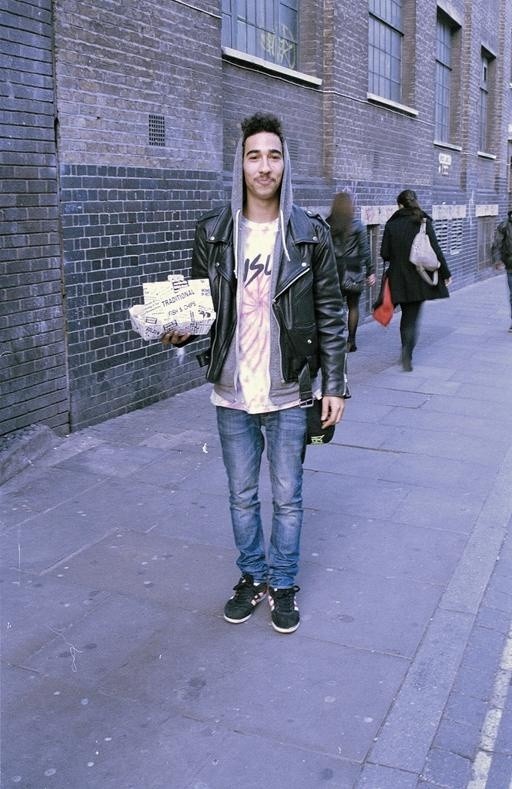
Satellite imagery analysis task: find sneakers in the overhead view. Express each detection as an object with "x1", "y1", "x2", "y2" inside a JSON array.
[
  {"x1": 223, "y1": 573, "x2": 268, "y2": 625},
  {"x1": 349, "y1": 345, "x2": 357, "y2": 352},
  {"x1": 267, "y1": 585, "x2": 301, "y2": 634},
  {"x1": 401, "y1": 343, "x2": 413, "y2": 373}
]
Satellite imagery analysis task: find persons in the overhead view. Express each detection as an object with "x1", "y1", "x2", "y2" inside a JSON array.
[
  {"x1": 158, "y1": 110, "x2": 352, "y2": 634},
  {"x1": 491, "y1": 209, "x2": 512, "y2": 333},
  {"x1": 380, "y1": 190, "x2": 453, "y2": 371},
  {"x1": 324, "y1": 193, "x2": 376, "y2": 354}
]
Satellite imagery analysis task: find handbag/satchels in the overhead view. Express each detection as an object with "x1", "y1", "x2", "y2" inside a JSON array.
[
  {"x1": 304, "y1": 399, "x2": 335, "y2": 445},
  {"x1": 409, "y1": 217, "x2": 445, "y2": 287},
  {"x1": 370, "y1": 260, "x2": 395, "y2": 327},
  {"x1": 340, "y1": 270, "x2": 365, "y2": 293}
]
